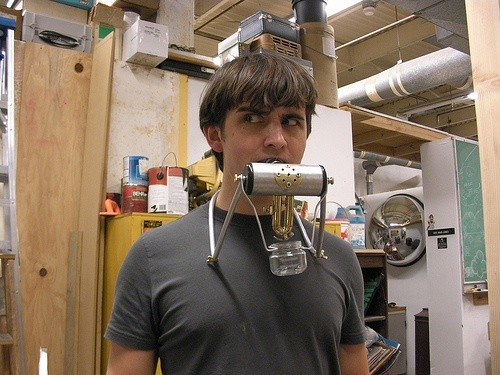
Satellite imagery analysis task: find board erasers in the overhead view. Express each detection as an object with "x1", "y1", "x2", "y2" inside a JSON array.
[{"x1": 467, "y1": 288, "x2": 481, "y2": 292}]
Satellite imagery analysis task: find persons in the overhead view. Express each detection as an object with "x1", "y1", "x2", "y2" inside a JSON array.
[{"x1": 104, "y1": 53, "x2": 370, "y2": 375}]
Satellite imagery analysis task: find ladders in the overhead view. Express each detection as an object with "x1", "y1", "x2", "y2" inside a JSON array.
[{"x1": 0, "y1": 16, "x2": 26, "y2": 375}]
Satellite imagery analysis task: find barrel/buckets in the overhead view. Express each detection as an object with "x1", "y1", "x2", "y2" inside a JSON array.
[
  {"x1": 148, "y1": 152, "x2": 189, "y2": 215},
  {"x1": 292, "y1": 0, "x2": 327, "y2": 25},
  {"x1": 311, "y1": 202, "x2": 350, "y2": 243},
  {"x1": 300, "y1": 23, "x2": 338, "y2": 108}
]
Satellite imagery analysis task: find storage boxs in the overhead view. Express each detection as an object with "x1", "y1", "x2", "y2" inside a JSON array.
[
  {"x1": 240, "y1": 10, "x2": 301, "y2": 44},
  {"x1": 121, "y1": 20, "x2": 169, "y2": 68},
  {"x1": 244, "y1": 33, "x2": 302, "y2": 59},
  {"x1": 218, "y1": 31, "x2": 240, "y2": 55}
]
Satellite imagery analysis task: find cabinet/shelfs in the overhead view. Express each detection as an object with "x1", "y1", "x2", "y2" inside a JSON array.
[
  {"x1": 353, "y1": 249, "x2": 388, "y2": 340},
  {"x1": 101, "y1": 212, "x2": 350, "y2": 375}
]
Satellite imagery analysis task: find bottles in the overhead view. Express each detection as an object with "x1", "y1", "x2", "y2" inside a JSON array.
[{"x1": 269, "y1": 241, "x2": 307, "y2": 276}]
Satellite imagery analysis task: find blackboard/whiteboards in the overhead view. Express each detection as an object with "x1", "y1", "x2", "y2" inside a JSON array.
[{"x1": 451, "y1": 137, "x2": 491, "y2": 284}]
